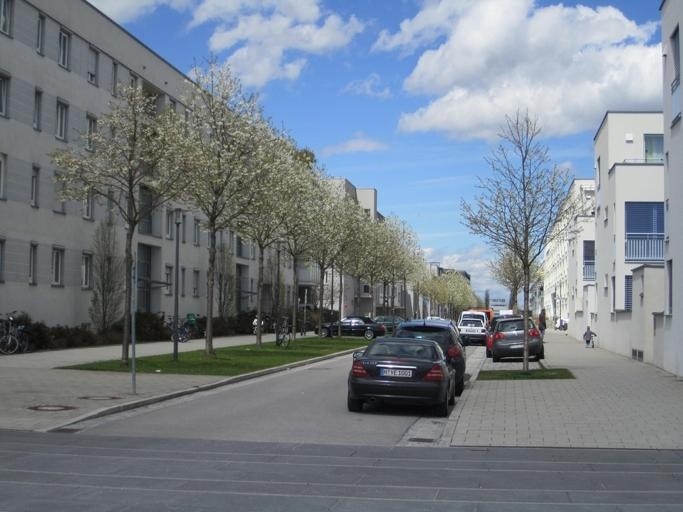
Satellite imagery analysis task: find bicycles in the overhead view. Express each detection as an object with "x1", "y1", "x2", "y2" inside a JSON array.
[
  {"x1": 275, "y1": 321, "x2": 297, "y2": 350},
  {"x1": 0, "y1": 310, "x2": 29, "y2": 355},
  {"x1": 160, "y1": 312, "x2": 193, "y2": 343}
]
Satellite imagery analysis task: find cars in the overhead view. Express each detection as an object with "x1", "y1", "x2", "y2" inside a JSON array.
[
  {"x1": 349, "y1": 315, "x2": 466, "y2": 417},
  {"x1": 457, "y1": 308, "x2": 544, "y2": 362},
  {"x1": 313, "y1": 315, "x2": 385, "y2": 340}
]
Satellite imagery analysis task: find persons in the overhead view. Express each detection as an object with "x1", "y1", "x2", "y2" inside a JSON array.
[
  {"x1": 250, "y1": 314, "x2": 264, "y2": 335},
  {"x1": 581, "y1": 325, "x2": 596, "y2": 347},
  {"x1": 537, "y1": 308, "x2": 549, "y2": 344}
]
